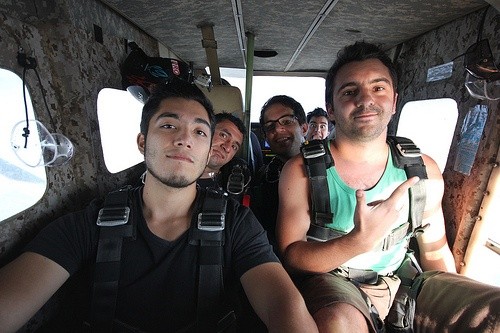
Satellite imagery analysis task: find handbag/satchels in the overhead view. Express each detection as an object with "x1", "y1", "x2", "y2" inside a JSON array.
[{"x1": 119, "y1": 42, "x2": 194, "y2": 91}]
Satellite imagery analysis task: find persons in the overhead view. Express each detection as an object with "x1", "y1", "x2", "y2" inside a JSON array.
[
  {"x1": 0, "y1": 81, "x2": 320, "y2": 333},
  {"x1": 275, "y1": 40, "x2": 500, "y2": 333},
  {"x1": 133, "y1": 95, "x2": 332, "y2": 242}
]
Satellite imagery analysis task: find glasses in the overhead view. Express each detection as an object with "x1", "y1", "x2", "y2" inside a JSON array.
[{"x1": 262, "y1": 115, "x2": 298, "y2": 132}]
